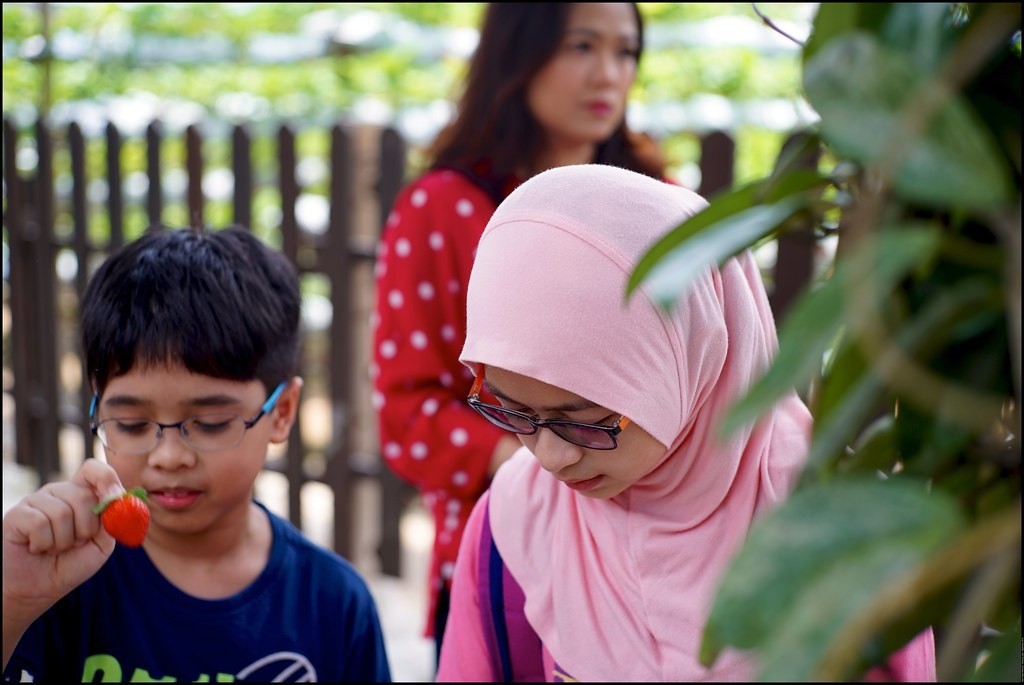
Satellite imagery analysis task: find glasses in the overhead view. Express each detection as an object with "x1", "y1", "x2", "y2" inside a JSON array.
[
  {"x1": 88, "y1": 381, "x2": 289, "y2": 454},
  {"x1": 467, "y1": 362, "x2": 633, "y2": 451}
]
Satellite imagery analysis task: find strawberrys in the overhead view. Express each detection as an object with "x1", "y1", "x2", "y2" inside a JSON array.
[{"x1": 93, "y1": 485, "x2": 152, "y2": 549}]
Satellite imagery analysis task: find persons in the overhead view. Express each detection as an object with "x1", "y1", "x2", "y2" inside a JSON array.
[
  {"x1": 369, "y1": 3, "x2": 695, "y2": 683},
  {"x1": 4, "y1": 223, "x2": 394, "y2": 684},
  {"x1": 428, "y1": 160, "x2": 944, "y2": 682}
]
